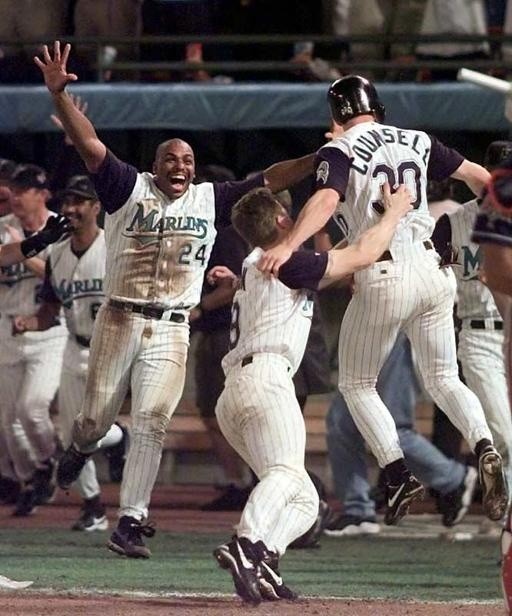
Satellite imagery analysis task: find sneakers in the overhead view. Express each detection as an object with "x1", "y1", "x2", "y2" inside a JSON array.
[
  {"x1": 0, "y1": 422, "x2": 129, "y2": 532},
  {"x1": 108, "y1": 518, "x2": 156, "y2": 559},
  {"x1": 0, "y1": 160, "x2": 16, "y2": 184},
  {"x1": 256, "y1": 546, "x2": 299, "y2": 602},
  {"x1": 213, "y1": 538, "x2": 262, "y2": 605},
  {"x1": 202, "y1": 484, "x2": 257, "y2": 510},
  {"x1": 324, "y1": 445, "x2": 509, "y2": 537}
]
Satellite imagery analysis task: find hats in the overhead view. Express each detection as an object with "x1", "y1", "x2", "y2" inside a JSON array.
[
  {"x1": 60, "y1": 176, "x2": 98, "y2": 199},
  {"x1": 9, "y1": 166, "x2": 50, "y2": 189}
]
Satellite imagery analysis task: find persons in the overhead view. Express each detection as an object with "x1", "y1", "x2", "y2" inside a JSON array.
[
  {"x1": 213, "y1": 181, "x2": 422, "y2": 606},
  {"x1": 0, "y1": 136, "x2": 512, "y2": 549},
  {"x1": 255, "y1": 72, "x2": 511, "y2": 527},
  {"x1": 31, "y1": 37, "x2": 351, "y2": 562},
  {"x1": 0, "y1": 1, "x2": 512, "y2": 83}
]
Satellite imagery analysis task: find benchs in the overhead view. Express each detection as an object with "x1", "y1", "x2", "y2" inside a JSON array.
[{"x1": 113, "y1": 401, "x2": 436, "y2": 496}]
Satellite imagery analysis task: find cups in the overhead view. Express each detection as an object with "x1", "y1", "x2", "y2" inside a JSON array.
[{"x1": 294, "y1": 40, "x2": 314, "y2": 60}]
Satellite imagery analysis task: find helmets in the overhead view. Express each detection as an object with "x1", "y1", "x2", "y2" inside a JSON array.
[{"x1": 327, "y1": 75, "x2": 386, "y2": 125}]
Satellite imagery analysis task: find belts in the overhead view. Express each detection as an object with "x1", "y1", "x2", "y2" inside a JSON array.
[
  {"x1": 374, "y1": 241, "x2": 433, "y2": 262},
  {"x1": 457, "y1": 319, "x2": 504, "y2": 330},
  {"x1": 109, "y1": 300, "x2": 192, "y2": 322},
  {"x1": 241, "y1": 356, "x2": 291, "y2": 371}
]
